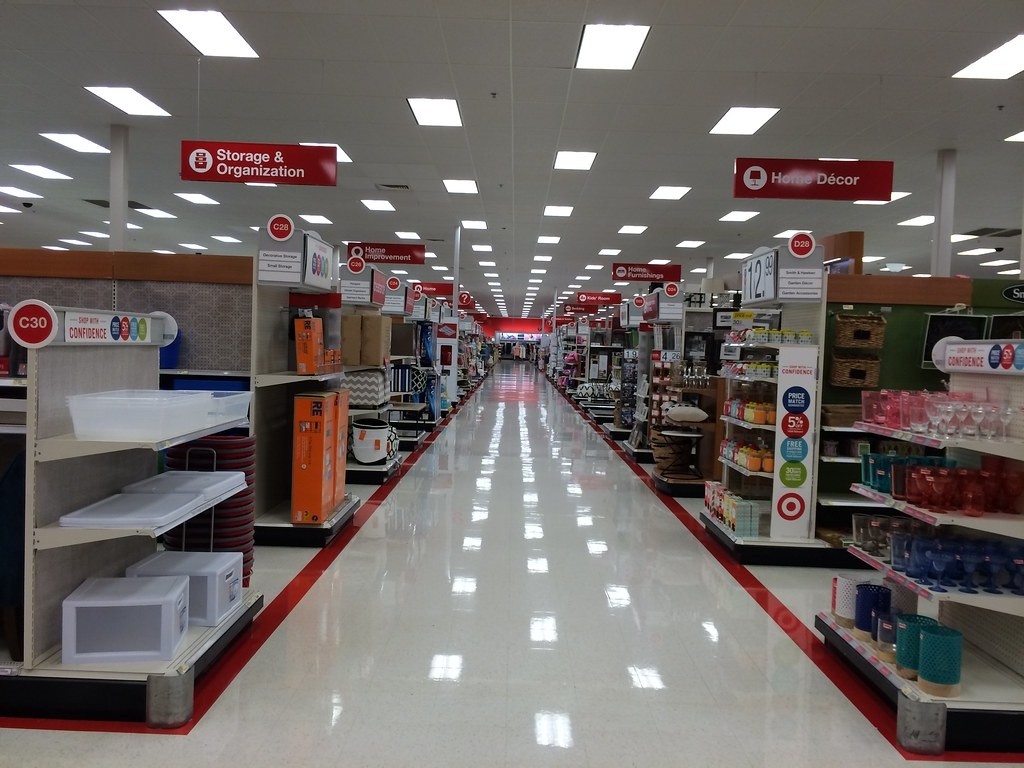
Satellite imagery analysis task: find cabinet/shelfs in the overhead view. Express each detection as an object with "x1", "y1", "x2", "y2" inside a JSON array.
[
  {"x1": 544, "y1": 308, "x2": 724, "y2": 498},
  {"x1": 814, "y1": 339, "x2": 1024, "y2": 755},
  {"x1": 700, "y1": 274, "x2": 1024, "y2": 565},
  {"x1": 0, "y1": 225, "x2": 362, "y2": 551},
  {"x1": 337, "y1": 261, "x2": 501, "y2": 487},
  {"x1": 0, "y1": 306, "x2": 265, "y2": 727}
]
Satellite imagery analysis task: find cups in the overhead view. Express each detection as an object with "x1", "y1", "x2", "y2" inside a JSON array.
[
  {"x1": 721, "y1": 362, "x2": 779, "y2": 378},
  {"x1": 724, "y1": 328, "x2": 813, "y2": 344},
  {"x1": 723, "y1": 398, "x2": 777, "y2": 426},
  {"x1": 860, "y1": 389, "x2": 1015, "y2": 441},
  {"x1": 830, "y1": 514, "x2": 1024, "y2": 596},
  {"x1": 861, "y1": 453, "x2": 1024, "y2": 517},
  {"x1": 720, "y1": 437, "x2": 774, "y2": 473}
]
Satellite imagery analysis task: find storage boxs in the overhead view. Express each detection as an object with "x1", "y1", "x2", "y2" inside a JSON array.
[
  {"x1": 294, "y1": 318, "x2": 325, "y2": 376},
  {"x1": 362, "y1": 312, "x2": 392, "y2": 367},
  {"x1": 324, "y1": 388, "x2": 351, "y2": 512},
  {"x1": 63, "y1": 389, "x2": 255, "y2": 444},
  {"x1": 291, "y1": 391, "x2": 336, "y2": 525},
  {"x1": 341, "y1": 313, "x2": 361, "y2": 367}
]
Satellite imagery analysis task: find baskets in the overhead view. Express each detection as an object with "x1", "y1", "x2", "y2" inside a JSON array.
[
  {"x1": 918, "y1": 625, "x2": 963, "y2": 697},
  {"x1": 895, "y1": 614, "x2": 938, "y2": 681},
  {"x1": 830, "y1": 574, "x2": 918, "y2": 651}
]
